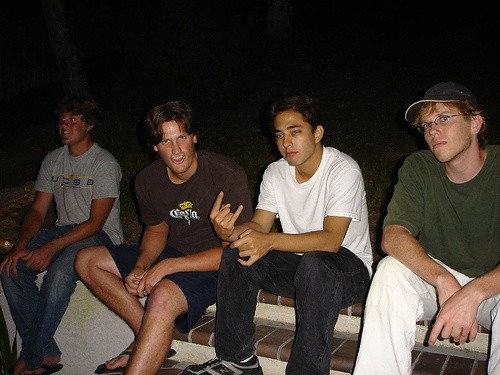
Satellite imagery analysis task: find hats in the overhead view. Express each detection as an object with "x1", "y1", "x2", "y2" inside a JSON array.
[{"x1": 405, "y1": 82, "x2": 478, "y2": 123}]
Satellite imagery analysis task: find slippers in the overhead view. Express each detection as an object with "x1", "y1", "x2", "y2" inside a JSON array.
[
  {"x1": 95, "y1": 349, "x2": 176, "y2": 374},
  {"x1": 7, "y1": 354, "x2": 22, "y2": 375},
  {"x1": 38, "y1": 363, "x2": 63, "y2": 375}
]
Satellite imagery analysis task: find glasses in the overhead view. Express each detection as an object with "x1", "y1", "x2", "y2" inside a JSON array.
[{"x1": 418, "y1": 114, "x2": 477, "y2": 133}]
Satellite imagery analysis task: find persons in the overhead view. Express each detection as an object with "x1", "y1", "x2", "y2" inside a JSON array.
[
  {"x1": 180, "y1": 94, "x2": 373, "y2": 375},
  {"x1": 74, "y1": 101, "x2": 254, "y2": 375},
  {"x1": 352, "y1": 84, "x2": 500, "y2": 375},
  {"x1": 0, "y1": 95, "x2": 125, "y2": 375}
]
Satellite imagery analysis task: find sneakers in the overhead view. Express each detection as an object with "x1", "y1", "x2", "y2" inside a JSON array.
[{"x1": 182, "y1": 354, "x2": 264, "y2": 375}]
